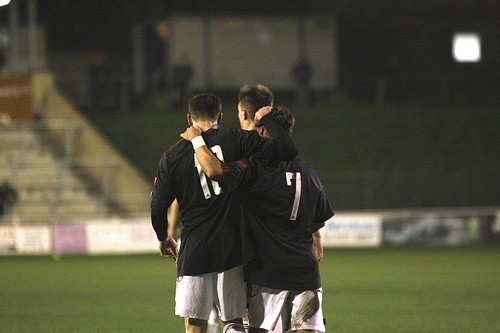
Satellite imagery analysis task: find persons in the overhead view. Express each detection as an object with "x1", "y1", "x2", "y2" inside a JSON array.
[
  {"x1": 219, "y1": 83, "x2": 324, "y2": 264},
  {"x1": 177, "y1": 107, "x2": 330, "y2": 333},
  {"x1": 151, "y1": 94, "x2": 297, "y2": 333}
]
